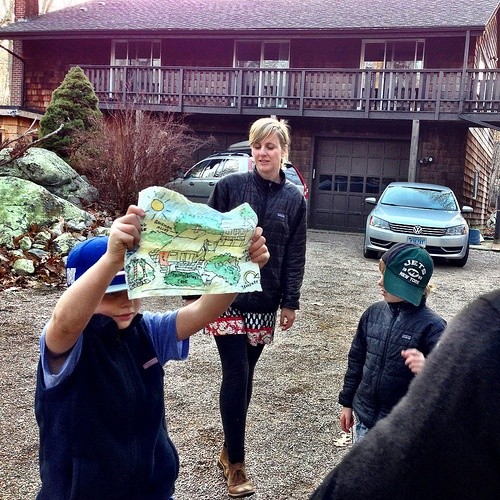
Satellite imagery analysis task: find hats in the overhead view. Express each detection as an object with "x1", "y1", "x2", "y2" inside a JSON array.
[
  {"x1": 382, "y1": 242, "x2": 434, "y2": 307},
  {"x1": 64, "y1": 237, "x2": 128, "y2": 296}
]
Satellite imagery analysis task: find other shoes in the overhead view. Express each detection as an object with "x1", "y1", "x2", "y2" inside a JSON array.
[
  {"x1": 226, "y1": 459, "x2": 255, "y2": 498},
  {"x1": 217, "y1": 445, "x2": 229, "y2": 478}
]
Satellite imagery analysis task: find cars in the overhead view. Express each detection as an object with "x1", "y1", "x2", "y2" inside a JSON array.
[{"x1": 363, "y1": 181, "x2": 474, "y2": 270}]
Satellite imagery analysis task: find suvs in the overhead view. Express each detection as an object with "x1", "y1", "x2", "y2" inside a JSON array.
[{"x1": 162, "y1": 148, "x2": 310, "y2": 224}]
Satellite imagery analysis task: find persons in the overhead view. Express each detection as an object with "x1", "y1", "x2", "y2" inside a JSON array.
[
  {"x1": 309, "y1": 290, "x2": 500, "y2": 499},
  {"x1": 182, "y1": 118, "x2": 307, "y2": 497},
  {"x1": 34, "y1": 205, "x2": 270, "y2": 500},
  {"x1": 338, "y1": 242, "x2": 447, "y2": 442}
]
